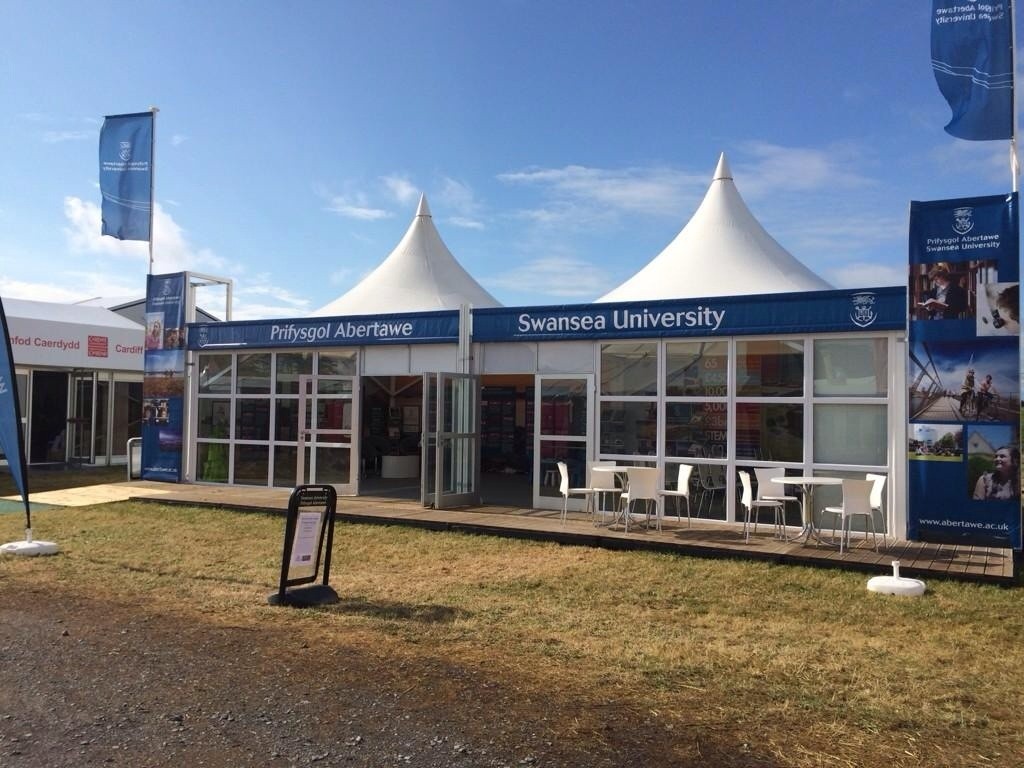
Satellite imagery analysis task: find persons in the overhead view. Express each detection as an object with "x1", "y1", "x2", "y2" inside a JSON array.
[
  {"x1": 915, "y1": 264, "x2": 1020, "y2": 501},
  {"x1": 203, "y1": 405, "x2": 231, "y2": 481},
  {"x1": 146, "y1": 321, "x2": 184, "y2": 349},
  {"x1": 144, "y1": 406, "x2": 167, "y2": 423}
]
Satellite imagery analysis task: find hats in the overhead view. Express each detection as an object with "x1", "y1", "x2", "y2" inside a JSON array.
[{"x1": 968, "y1": 369, "x2": 975, "y2": 373}]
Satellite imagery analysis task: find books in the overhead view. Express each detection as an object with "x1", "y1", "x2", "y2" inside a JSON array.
[{"x1": 914, "y1": 259, "x2": 976, "y2": 319}]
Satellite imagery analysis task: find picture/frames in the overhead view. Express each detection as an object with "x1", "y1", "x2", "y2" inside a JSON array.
[{"x1": 212, "y1": 401, "x2": 231, "y2": 426}]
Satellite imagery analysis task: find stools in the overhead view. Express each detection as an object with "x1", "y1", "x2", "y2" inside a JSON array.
[{"x1": 544, "y1": 470, "x2": 561, "y2": 488}]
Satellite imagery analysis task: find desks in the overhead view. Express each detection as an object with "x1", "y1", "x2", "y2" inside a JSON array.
[
  {"x1": 592, "y1": 465, "x2": 646, "y2": 532},
  {"x1": 771, "y1": 476, "x2": 845, "y2": 548}
]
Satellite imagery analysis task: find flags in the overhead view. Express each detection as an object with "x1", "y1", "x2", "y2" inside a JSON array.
[
  {"x1": 99, "y1": 112, "x2": 153, "y2": 241},
  {"x1": 929, "y1": 0, "x2": 1013, "y2": 141},
  {"x1": 0, "y1": 315, "x2": 24, "y2": 503}
]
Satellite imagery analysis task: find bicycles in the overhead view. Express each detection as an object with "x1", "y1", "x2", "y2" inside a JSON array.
[{"x1": 961, "y1": 388, "x2": 995, "y2": 423}]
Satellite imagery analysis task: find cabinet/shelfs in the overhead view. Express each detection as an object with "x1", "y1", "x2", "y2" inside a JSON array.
[{"x1": 909, "y1": 261, "x2": 999, "y2": 321}]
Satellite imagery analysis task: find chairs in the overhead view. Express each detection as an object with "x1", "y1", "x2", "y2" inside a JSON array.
[
  {"x1": 739, "y1": 468, "x2": 802, "y2": 544},
  {"x1": 634, "y1": 451, "x2": 697, "y2": 511},
  {"x1": 696, "y1": 447, "x2": 772, "y2": 518},
  {"x1": 815, "y1": 472, "x2": 888, "y2": 555},
  {"x1": 556, "y1": 460, "x2": 694, "y2": 536}
]
